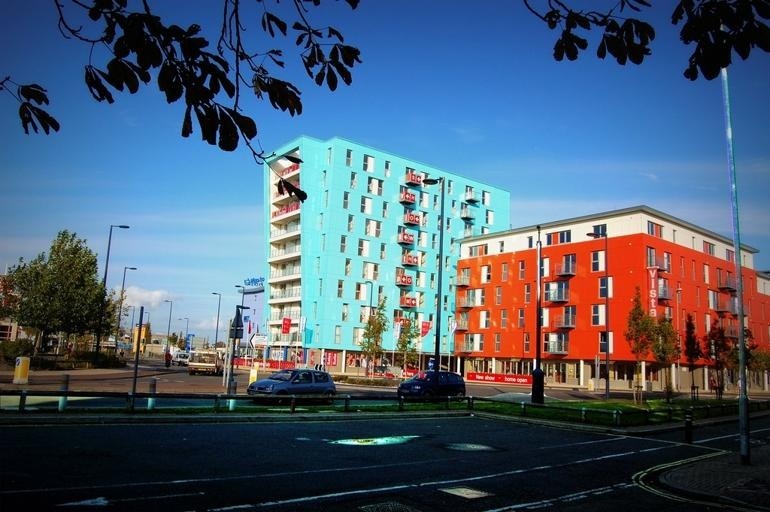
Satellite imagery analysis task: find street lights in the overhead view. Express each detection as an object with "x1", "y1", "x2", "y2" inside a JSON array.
[
  {"x1": 116, "y1": 267, "x2": 150, "y2": 348},
  {"x1": 532, "y1": 225, "x2": 544, "y2": 402},
  {"x1": 422, "y1": 176, "x2": 445, "y2": 372},
  {"x1": 677, "y1": 288, "x2": 682, "y2": 394},
  {"x1": 212, "y1": 285, "x2": 250, "y2": 394},
  {"x1": 163, "y1": 299, "x2": 172, "y2": 346},
  {"x1": 95, "y1": 224, "x2": 129, "y2": 354},
  {"x1": 587, "y1": 232, "x2": 610, "y2": 399},
  {"x1": 184, "y1": 317, "x2": 188, "y2": 337},
  {"x1": 364, "y1": 280, "x2": 374, "y2": 375}
]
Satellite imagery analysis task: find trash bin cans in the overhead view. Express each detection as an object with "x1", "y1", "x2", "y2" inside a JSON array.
[
  {"x1": 13, "y1": 358, "x2": 29, "y2": 384},
  {"x1": 588, "y1": 379, "x2": 595, "y2": 391},
  {"x1": 646, "y1": 382, "x2": 652, "y2": 393}
]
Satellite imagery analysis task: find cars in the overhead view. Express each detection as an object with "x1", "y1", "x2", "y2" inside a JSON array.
[
  {"x1": 246, "y1": 369, "x2": 336, "y2": 405},
  {"x1": 397, "y1": 371, "x2": 466, "y2": 402},
  {"x1": 172, "y1": 352, "x2": 188, "y2": 367}
]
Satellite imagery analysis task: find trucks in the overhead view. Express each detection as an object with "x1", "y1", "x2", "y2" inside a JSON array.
[{"x1": 188, "y1": 350, "x2": 223, "y2": 375}]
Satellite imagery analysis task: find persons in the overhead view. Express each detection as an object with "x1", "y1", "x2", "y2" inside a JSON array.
[
  {"x1": 165, "y1": 350, "x2": 173, "y2": 369},
  {"x1": 315, "y1": 364, "x2": 322, "y2": 371},
  {"x1": 120, "y1": 349, "x2": 124, "y2": 358},
  {"x1": 139, "y1": 350, "x2": 144, "y2": 366}
]
